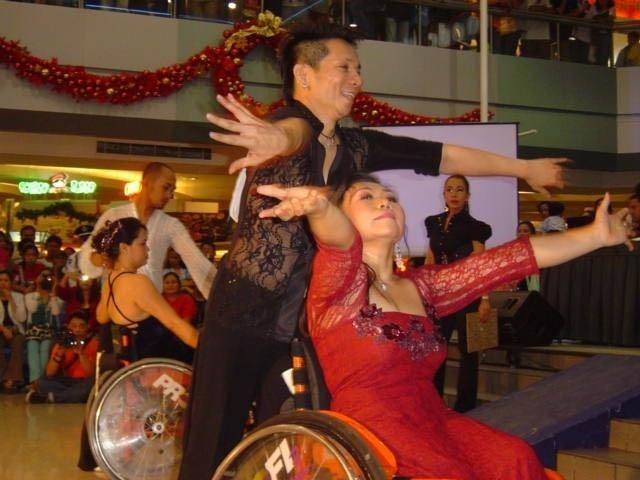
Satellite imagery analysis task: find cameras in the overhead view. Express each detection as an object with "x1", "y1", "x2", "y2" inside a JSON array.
[{"x1": 57, "y1": 329, "x2": 76, "y2": 348}]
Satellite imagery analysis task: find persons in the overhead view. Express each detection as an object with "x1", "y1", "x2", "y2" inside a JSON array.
[
  {"x1": 125, "y1": 0, "x2": 640, "y2": 72},
  {"x1": 180, "y1": 24, "x2": 575, "y2": 480},
  {"x1": 0, "y1": 223, "x2": 222, "y2": 406},
  {"x1": 512, "y1": 194, "x2": 639, "y2": 294},
  {"x1": 74, "y1": 158, "x2": 220, "y2": 473},
  {"x1": 92, "y1": 213, "x2": 204, "y2": 366},
  {"x1": 419, "y1": 175, "x2": 494, "y2": 413},
  {"x1": 247, "y1": 163, "x2": 640, "y2": 478}
]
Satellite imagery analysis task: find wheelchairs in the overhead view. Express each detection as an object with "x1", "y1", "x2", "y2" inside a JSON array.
[
  {"x1": 66, "y1": 317, "x2": 193, "y2": 480},
  {"x1": 206, "y1": 299, "x2": 396, "y2": 480}
]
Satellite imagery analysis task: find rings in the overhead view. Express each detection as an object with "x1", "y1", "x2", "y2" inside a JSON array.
[{"x1": 623, "y1": 222, "x2": 629, "y2": 228}]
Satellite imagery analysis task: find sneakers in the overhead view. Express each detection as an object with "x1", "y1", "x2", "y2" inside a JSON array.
[{"x1": 26, "y1": 388, "x2": 40, "y2": 405}]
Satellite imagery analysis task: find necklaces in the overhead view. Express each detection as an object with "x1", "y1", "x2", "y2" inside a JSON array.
[{"x1": 369, "y1": 270, "x2": 400, "y2": 292}]
